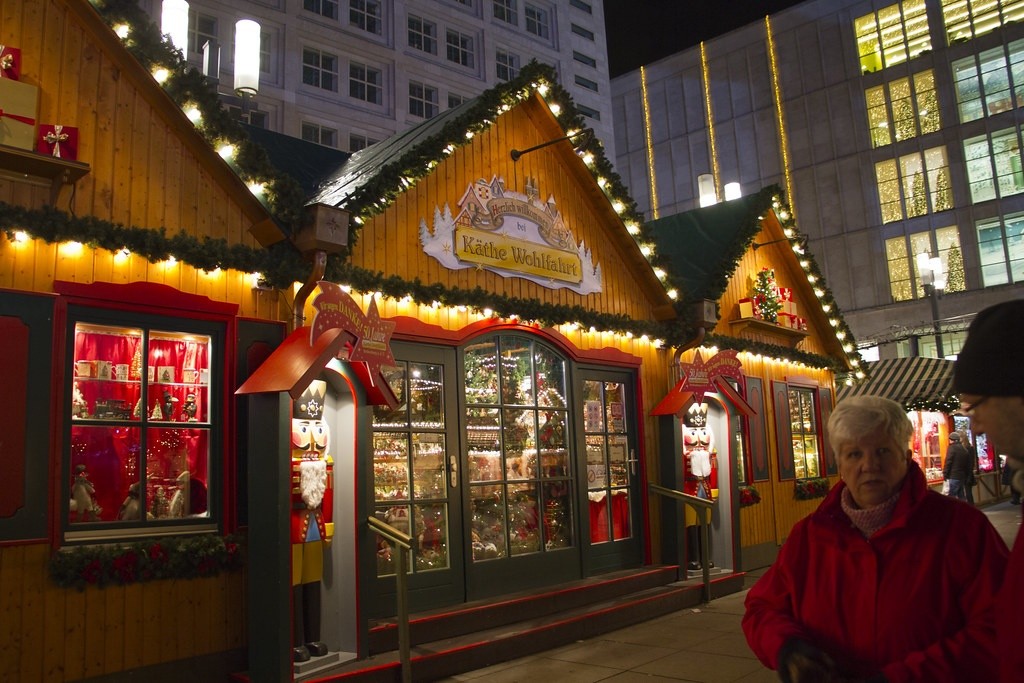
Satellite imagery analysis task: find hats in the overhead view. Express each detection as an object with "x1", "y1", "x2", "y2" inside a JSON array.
[
  {"x1": 949, "y1": 432, "x2": 960, "y2": 440},
  {"x1": 954, "y1": 298, "x2": 1024, "y2": 396}
]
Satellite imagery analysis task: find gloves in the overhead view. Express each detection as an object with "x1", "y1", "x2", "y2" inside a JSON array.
[{"x1": 783, "y1": 641, "x2": 837, "y2": 683}]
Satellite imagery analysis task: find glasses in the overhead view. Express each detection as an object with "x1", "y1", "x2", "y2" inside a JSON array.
[{"x1": 959, "y1": 396, "x2": 989, "y2": 421}]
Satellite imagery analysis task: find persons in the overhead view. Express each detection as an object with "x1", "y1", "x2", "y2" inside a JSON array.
[
  {"x1": 942, "y1": 430, "x2": 976, "y2": 504},
  {"x1": 290, "y1": 380, "x2": 335, "y2": 663},
  {"x1": 682, "y1": 400, "x2": 720, "y2": 570},
  {"x1": 951, "y1": 297, "x2": 1024, "y2": 683},
  {"x1": 741, "y1": 395, "x2": 1013, "y2": 683}
]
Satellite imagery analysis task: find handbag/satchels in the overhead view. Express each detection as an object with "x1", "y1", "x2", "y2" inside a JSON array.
[
  {"x1": 941, "y1": 479, "x2": 950, "y2": 496},
  {"x1": 964, "y1": 475, "x2": 977, "y2": 486}
]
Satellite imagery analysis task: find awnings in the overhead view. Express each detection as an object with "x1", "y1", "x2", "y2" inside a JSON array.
[{"x1": 834, "y1": 356, "x2": 959, "y2": 408}]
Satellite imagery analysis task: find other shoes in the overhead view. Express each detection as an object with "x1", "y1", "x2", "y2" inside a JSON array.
[{"x1": 1010, "y1": 500, "x2": 1019, "y2": 505}]
